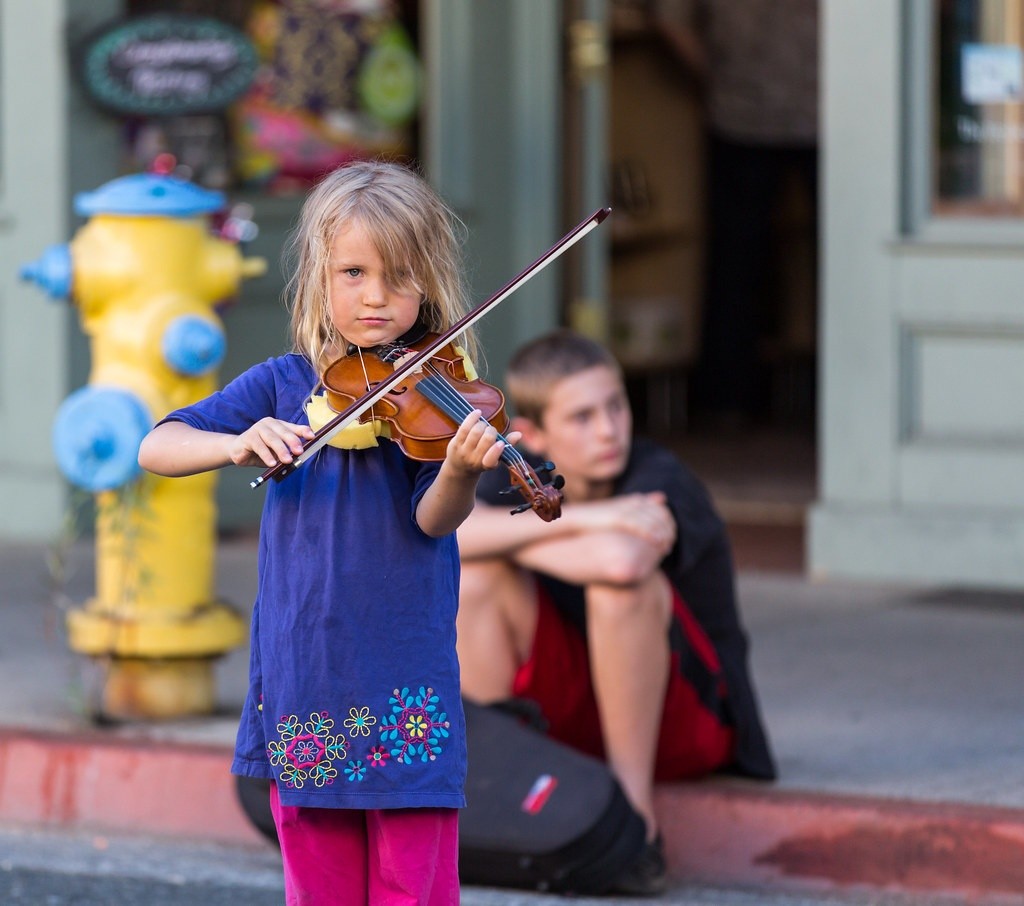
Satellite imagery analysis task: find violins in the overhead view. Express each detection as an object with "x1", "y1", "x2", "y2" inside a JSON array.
[{"x1": 321, "y1": 332, "x2": 566, "y2": 522}]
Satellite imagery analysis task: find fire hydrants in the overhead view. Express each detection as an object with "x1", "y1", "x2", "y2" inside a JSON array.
[{"x1": 18, "y1": 171, "x2": 252, "y2": 727}]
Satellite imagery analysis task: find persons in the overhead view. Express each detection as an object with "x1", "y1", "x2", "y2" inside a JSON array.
[
  {"x1": 138, "y1": 160, "x2": 524, "y2": 906},
  {"x1": 456, "y1": 337, "x2": 779, "y2": 898}
]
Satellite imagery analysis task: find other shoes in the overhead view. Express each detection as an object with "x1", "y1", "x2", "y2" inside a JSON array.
[{"x1": 608, "y1": 827, "x2": 674, "y2": 894}]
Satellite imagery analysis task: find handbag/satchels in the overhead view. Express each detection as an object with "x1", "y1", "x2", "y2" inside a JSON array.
[{"x1": 233, "y1": 690, "x2": 647, "y2": 894}]
